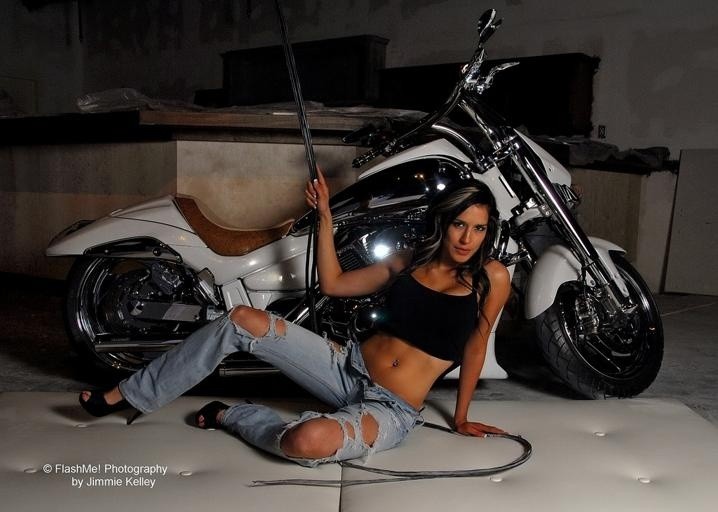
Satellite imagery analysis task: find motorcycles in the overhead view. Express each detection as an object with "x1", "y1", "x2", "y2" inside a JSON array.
[{"x1": 41, "y1": 9, "x2": 664, "y2": 399}]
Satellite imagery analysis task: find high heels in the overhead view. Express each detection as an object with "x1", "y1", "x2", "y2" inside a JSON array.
[
  {"x1": 79, "y1": 391, "x2": 142, "y2": 425},
  {"x1": 195, "y1": 398, "x2": 254, "y2": 429}
]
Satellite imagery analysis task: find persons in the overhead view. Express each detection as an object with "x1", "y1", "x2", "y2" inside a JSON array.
[{"x1": 78, "y1": 162, "x2": 511, "y2": 468}]
